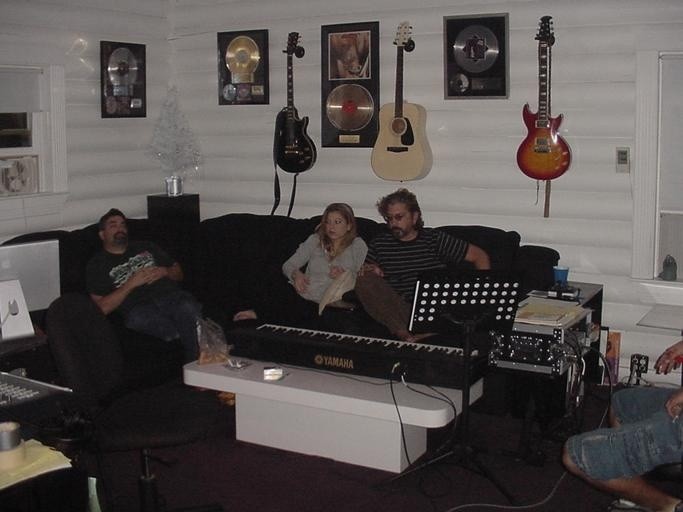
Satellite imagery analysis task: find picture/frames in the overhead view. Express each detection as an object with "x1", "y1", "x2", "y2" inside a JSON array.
[
  {"x1": 217, "y1": 29, "x2": 271, "y2": 106},
  {"x1": 443, "y1": 14, "x2": 510, "y2": 100},
  {"x1": 99, "y1": 39, "x2": 149, "y2": 119},
  {"x1": 321, "y1": 19, "x2": 381, "y2": 149}
]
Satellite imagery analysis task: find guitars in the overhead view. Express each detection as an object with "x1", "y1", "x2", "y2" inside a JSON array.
[
  {"x1": 371, "y1": 22, "x2": 434, "y2": 180},
  {"x1": 516, "y1": 16, "x2": 571, "y2": 180},
  {"x1": 274, "y1": 32, "x2": 316, "y2": 173}
]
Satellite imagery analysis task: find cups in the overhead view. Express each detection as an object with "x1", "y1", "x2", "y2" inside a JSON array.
[
  {"x1": 166, "y1": 175, "x2": 183, "y2": 197},
  {"x1": 0, "y1": 421, "x2": 21, "y2": 451},
  {"x1": 552, "y1": 265, "x2": 568, "y2": 284}
]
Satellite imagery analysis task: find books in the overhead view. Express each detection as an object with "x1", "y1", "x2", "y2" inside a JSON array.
[
  {"x1": 0, "y1": 438, "x2": 72, "y2": 490},
  {"x1": 512, "y1": 295, "x2": 585, "y2": 328}
]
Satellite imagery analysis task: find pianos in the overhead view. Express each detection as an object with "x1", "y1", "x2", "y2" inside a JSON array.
[{"x1": 231, "y1": 319, "x2": 482, "y2": 389}]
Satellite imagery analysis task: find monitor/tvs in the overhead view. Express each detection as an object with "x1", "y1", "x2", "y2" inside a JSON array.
[{"x1": 0, "y1": 239, "x2": 61, "y2": 341}]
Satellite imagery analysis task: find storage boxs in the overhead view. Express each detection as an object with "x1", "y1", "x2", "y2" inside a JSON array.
[{"x1": 147, "y1": 192, "x2": 200, "y2": 222}]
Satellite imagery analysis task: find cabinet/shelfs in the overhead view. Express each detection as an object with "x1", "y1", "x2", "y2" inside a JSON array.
[{"x1": 488, "y1": 281, "x2": 604, "y2": 467}]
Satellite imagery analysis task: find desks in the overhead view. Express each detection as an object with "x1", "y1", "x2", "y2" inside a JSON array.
[{"x1": 183, "y1": 320, "x2": 484, "y2": 474}]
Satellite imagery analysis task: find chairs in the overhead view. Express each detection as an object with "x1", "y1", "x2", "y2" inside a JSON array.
[{"x1": 644, "y1": 461, "x2": 683, "y2": 482}]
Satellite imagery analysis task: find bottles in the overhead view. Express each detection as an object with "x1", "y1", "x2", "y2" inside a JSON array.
[{"x1": 662, "y1": 255, "x2": 678, "y2": 280}]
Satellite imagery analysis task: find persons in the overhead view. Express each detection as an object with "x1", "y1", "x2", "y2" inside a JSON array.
[
  {"x1": 82, "y1": 207, "x2": 213, "y2": 392},
  {"x1": 354, "y1": 188, "x2": 493, "y2": 338},
  {"x1": 231, "y1": 201, "x2": 369, "y2": 323},
  {"x1": 560, "y1": 340, "x2": 682, "y2": 511}
]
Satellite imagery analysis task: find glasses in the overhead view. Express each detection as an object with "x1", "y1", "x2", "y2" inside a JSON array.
[{"x1": 385, "y1": 212, "x2": 408, "y2": 220}]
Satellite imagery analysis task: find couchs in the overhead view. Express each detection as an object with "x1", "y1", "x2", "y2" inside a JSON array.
[{"x1": 1, "y1": 214, "x2": 561, "y2": 509}]
